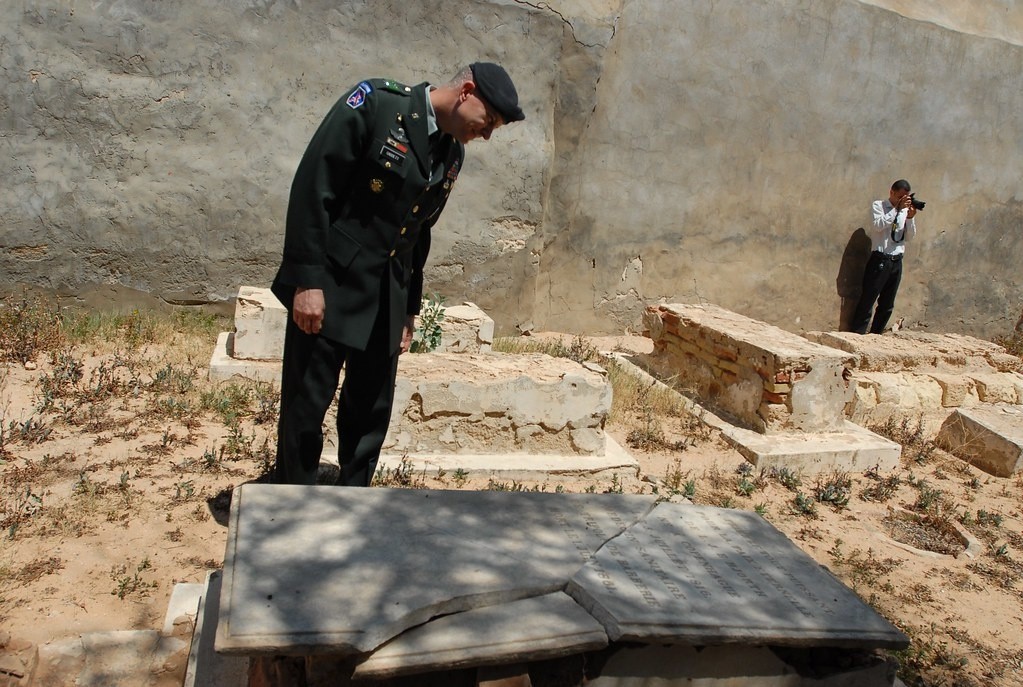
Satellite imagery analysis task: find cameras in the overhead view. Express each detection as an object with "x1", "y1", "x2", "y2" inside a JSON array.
[{"x1": 907, "y1": 193, "x2": 926, "y2": 211}]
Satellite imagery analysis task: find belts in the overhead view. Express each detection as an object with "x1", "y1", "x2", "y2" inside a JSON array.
[{"x1": 874, "y1": 251, "x2": 902, "y2": 260}]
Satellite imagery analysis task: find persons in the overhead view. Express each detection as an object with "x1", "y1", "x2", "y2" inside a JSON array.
[
  {"x1": 849, "y1": 180, "x2": 916, "y2": 334},
  {"x1": 270, "y1": 62, "x2": 525, "y2": 487}
]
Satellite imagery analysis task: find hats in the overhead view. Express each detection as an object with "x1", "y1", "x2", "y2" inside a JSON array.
[{"x1": 468, "y1": 62, "x2": 525, "y2": 124}]
